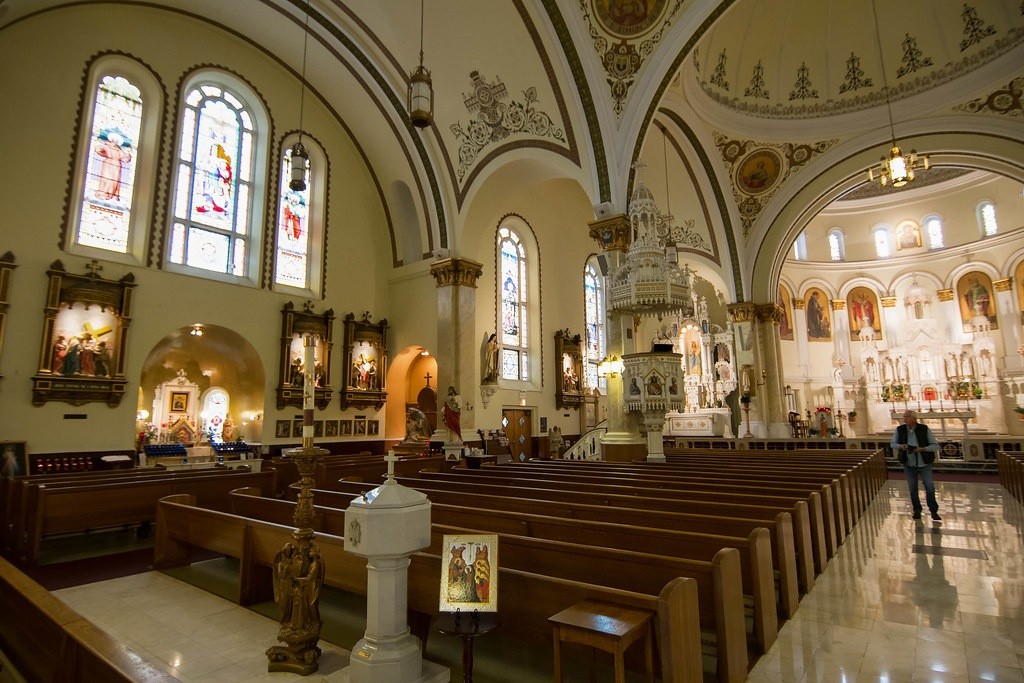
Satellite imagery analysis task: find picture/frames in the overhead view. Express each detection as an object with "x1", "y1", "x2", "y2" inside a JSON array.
[
  {"x1": 354, "y1": 420, "x2": 366, "y2": 435},
  {"x1": 171, "y1": 393, "x2": 188, "y2": 411},
  {"x1": 368, "y1": 420, "x2": 379, "y2": 435},
  {"x1": 340, "y1": 420, "x2": 352, "y2": 436},
  {"x1": 293, "y1": 420, "x2": 304, "y2": 437},
  {"x1": 314, "y1": 420, "x2": 324, "y2": 437},
  {"x1": 276, "y1": 420, "x2": 291, "y2": 438},
  {"x1": 325, "y1": 420, "x2": 338, "y2": 437},
  {"x1": 439, "y1": 534, "x2": 498, "y2": 613}
]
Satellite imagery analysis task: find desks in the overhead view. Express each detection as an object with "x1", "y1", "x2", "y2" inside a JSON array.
[{"x1": 466, "y1": 455, "x2": 498, "y2": 470}]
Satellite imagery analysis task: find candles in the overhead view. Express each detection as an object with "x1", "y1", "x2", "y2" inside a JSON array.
[
  {"x1": 905, "y1": 391, "x2": 909, "y2": 400},
  {"x1": 890, "y1": 392, "x2": 894, "y2": 402},
  {"x1": 917, "y1": 392, "x2": 920, "y2": 402},
  {"x1": 939, "y1": 391, "x2": 944, "y2": 402},
  {"x1": 966, "y1": 393, "x2": 970, "y2": 404},
  {"x1": 952, "y1": 390, "x2": 957, "y2": 404},
  {"x1": 928, "y1": 391, "x2": 932, "y2": 403}
]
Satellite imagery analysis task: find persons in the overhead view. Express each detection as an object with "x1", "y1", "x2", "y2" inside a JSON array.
[
  {"x1": 548, "y1": 426, "x2": 563, "y2": 458},
  {"x1": 289, "y1": 357, "x2": 326, "y2": 387},
  {"x1": 405, "y1": 408, "x2": 432, "y2": 442},
  {"x1": 565, "y1": 367, "x2": 578, "y2": 391},
  {"x1": 222, "y1": 412, "x2": 234, "y2": 443},
  {"x1": 891, "y1": 410, "x2": 943, "y2": 520},
  {"x1": 481, "y1": 334, "x2": 501, "y2": 384},
  {"x1": 688, "y1": 341, "x2": 702, "y2": 375},
  {"x1": 52, "y1": 333, "x2": 111, "y2": 378},
  {"x1": 439, "y1": 385, "x2": 463, "y2": 442},
  {"x1": 352, "y1": 354, "x2": 378, "y2": 391},
  {"x1": 819, "y1": 414, "x2": 828, "y2": 437}
]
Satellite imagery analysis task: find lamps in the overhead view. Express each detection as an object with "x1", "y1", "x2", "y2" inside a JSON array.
[
  {"x1": 406, "y1": 0, "x2": 435, "y2": 131},
  {"x1": 603, "y1": 354, "x2": 619, "y2": 379},
  {"x1": 288, "y1": 0, "x2": 309, "y2": 192},
  {"x1": 1015, "y1": 393, "x2": 1024, "y2": 409},
  {"x1": 866, "y1": 0, "x2": 933, "y2": 188}
]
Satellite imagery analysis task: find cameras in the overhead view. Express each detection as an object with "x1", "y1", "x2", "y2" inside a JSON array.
[{"x1": 907, "y1": 444, "x2": 917, "y2": 454}]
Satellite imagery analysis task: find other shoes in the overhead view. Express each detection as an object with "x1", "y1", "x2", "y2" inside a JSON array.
[
  {"x1": 913, "y1": 510, "x2": 922, "y2": 520},
  {"x1": 930, "y1": 511, "x2": 942, "y2": 521}
]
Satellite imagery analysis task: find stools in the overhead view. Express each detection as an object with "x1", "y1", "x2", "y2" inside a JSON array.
[
  {"x1": 548, "y1": 599, "x2": 653, "y2": 683},
  {"x1": 434, "y1": 614, "x2": 501, "y2": 683}
]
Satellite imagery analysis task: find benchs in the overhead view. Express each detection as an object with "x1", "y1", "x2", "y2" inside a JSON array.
[
  {"x1": 5, "y1": 464, "x2": 166, "y2": 547},
  {"x1": 27, "y1": 468, "x2": 277, "y2": 564},
  {"x1": 13, "y1": 465, "x2": 251, "y2": 547},
  {"x1": 154, "y1": 446, "x2": 890, "y2": 683},
  {"x1": 9, "y1": 463, "x2": 229, "y2": 543}
]
